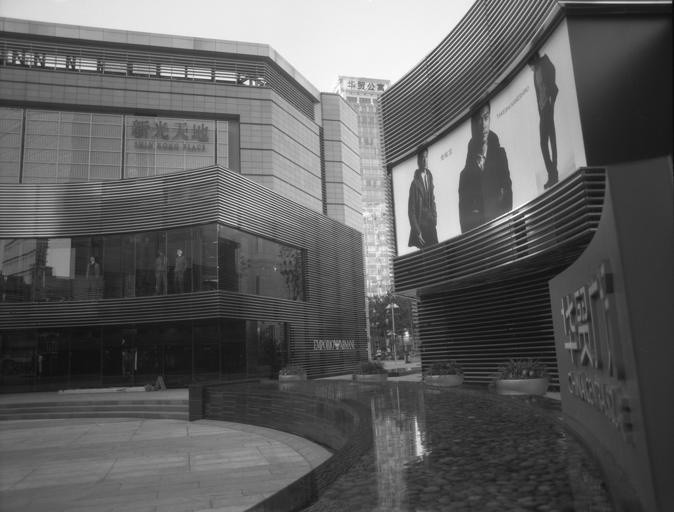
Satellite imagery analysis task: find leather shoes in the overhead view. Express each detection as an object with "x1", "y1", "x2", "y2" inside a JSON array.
[{"x1": 544, "y1": 177, "x2": 558, "y2": 189}]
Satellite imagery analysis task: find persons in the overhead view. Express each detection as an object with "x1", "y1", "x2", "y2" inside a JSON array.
[
  {"x1": 85, "y1": 256, "x2": 100, "y2": 298},
  {"x1": 408, "y1": 148, "x2": 438, "y2": 249},
  {"x1": 528, "y1": 52, "x2": 558, "y2": 189},
  {"x1": 458, "y1": 102, "x2": 514, "y2": 234},
  {"x1": 155, "y1": 249, "x2": 187, "y2": 295}
]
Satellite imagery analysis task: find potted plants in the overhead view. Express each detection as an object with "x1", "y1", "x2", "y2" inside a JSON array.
[
  {"x1": 278, "y1": 362, "x2": 307, "y2": 381},
  {"x1": 423, "y1": 359, "x2": 465, "y2": 387},
  {"x1": 353, "y1": 360, "x2": 388, "y2": 383},
  {"x1": 494, "y1": 356, "x2": 549, "y2": 397}
]
